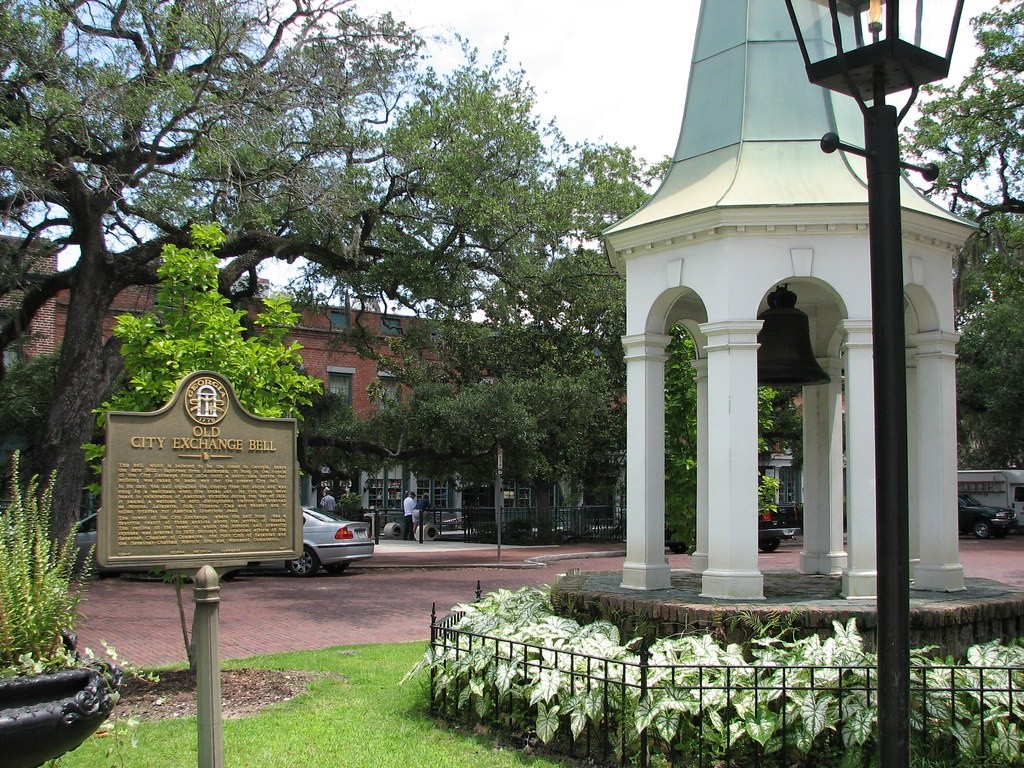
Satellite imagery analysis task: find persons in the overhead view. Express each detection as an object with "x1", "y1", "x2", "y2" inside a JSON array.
[
  {"x1": 402, "y1": 491, "x2": 417, "y2": 541},
  {"x1": 411, "y1": 493, "x2": 433, "y2": 541},
  {"x1": 319, "y1": 487, "x2": 336, "y2": 514}
]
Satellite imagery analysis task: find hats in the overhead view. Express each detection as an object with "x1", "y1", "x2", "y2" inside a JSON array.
[{"x1": 408, "y1": 492, "x2": 416, "y2": 496}]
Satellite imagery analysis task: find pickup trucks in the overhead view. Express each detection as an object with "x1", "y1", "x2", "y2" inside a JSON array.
[{"x1": 664, "y1": 503, "x2": 801, "y2": 555}]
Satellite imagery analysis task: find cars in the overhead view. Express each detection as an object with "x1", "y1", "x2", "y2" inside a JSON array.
[
  {"x1": 223, "y1": 509, "x2": 376, "y2": 578},
  {"x1": 72, "y1": 515, "x2": 121, "y2": 578},
  {"x1": 956, "y1": 494, "x2": 1017, "y2": 539}
]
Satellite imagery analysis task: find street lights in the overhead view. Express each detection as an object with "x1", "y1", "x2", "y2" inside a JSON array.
[{"x1": 785, "y1": 0, "x2": 965, "y2": 768}]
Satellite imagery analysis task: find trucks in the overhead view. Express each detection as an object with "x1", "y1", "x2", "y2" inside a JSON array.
[{"x1": 957, "y1": 469, "x2": 1024, "y2": 530}]
